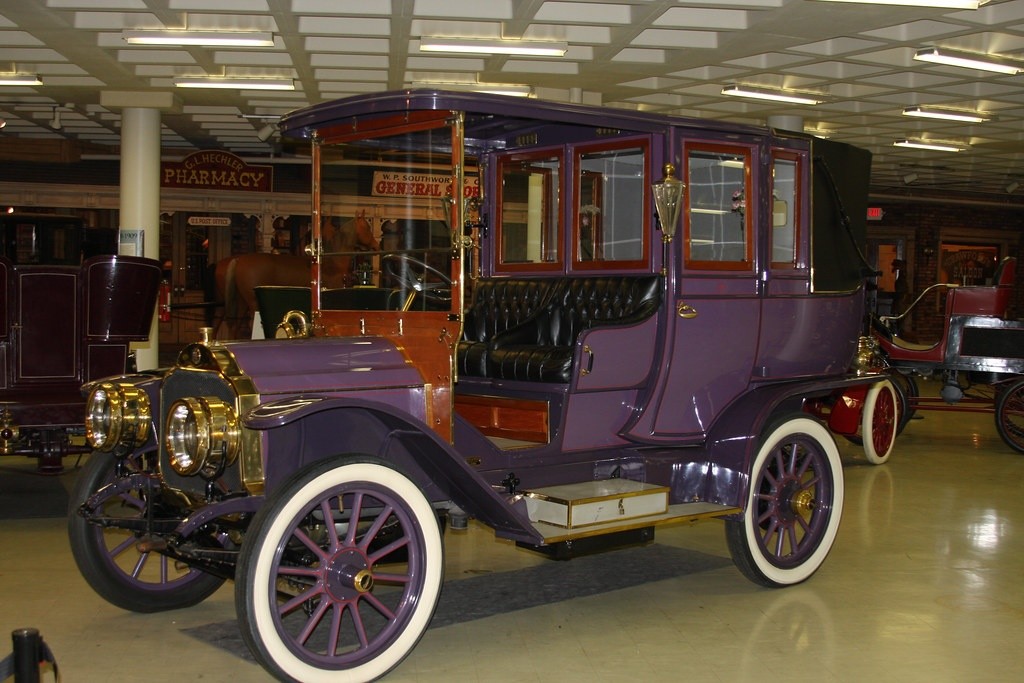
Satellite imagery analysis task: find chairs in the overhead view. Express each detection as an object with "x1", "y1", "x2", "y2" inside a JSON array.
[
  {"x1": 945, "y1": 256, "x2": 1017, "y2": 316},
  {"x1": 458, "y1": 273, "x2": 662, "y2": 402}
]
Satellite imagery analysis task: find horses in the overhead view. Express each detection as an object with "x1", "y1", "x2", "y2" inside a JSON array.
[{"x1": 202, "y1": 209, "x2": 381, "y2": 339}]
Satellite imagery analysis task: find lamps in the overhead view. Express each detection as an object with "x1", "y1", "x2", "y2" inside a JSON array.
[
  {"x1": 902, "y1": 108, "x2": 994, "y2": 123},
  {"x1": 440, "y1": 192, "x2": 488, "y2": 242},
  {"x1": 913, "y1": 49, "x2": 1024, "y2": 74},
  {"x1": 171, "y1": 77, "x2": 295, "y2": 91},
  {"x1": 721, "y1": 85, "x2": 819, "y2": 106},
  {"x1": 257, "y1": 123, "x2": 275, "y2": 141},
  {"x1": 893, "y1": 140, "x2": 970, "y2": 152},
  {"x1": 121, "y1": 32, "x2": 274, "y2": 46},
  {"x1": 48, "y1": 110, "x2": 61, "y2": 130},
  {"x1": 410, "y1": 82, "x2": 531, "y2": 97},
  {"x1": 0, "y1": 76, "x2": 44, "y2": 86},
  {"x1": 652, "y1": 163, "x2": 685, "y2": 244},
  {"x1": 419, "y1": 37, "x2": 569, "y2": 57}
]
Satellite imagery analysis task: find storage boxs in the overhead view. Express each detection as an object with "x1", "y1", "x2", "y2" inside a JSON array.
[{"x1": 518, "y1": 478, "x2": 670, "y2": 530}]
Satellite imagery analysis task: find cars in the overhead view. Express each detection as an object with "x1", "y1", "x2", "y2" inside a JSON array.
[{"x1": 61, "y1": 84, "x2": 888, "y2": 682}]
